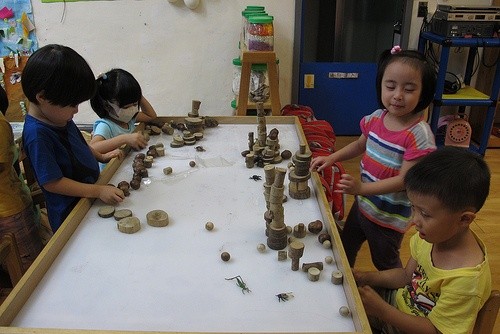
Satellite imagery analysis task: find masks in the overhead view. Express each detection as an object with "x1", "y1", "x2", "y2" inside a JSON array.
[{"x1": 103, "y1": 100, "x2": 137, "y2": 124}]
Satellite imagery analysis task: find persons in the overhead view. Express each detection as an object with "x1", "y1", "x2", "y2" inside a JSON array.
[
  {"x1": 23, "y1": 45, "x2": 126, "y2": 235},
  {"x1": 311, "y1": 45, "x2": 438, "y2": 270},
  {"x1": 91, "y1": 68, "x2": 157, "y2": 154},
  {"x1": 0, "y1": 84, "x2": 53, "y2": 288},
  {"x1": 352, "y1": 145, "x2": 491, "y2": 334}
]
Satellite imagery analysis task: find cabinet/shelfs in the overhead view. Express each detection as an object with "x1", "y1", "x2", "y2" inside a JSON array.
[
  {"x1": 419, "y1": 31, "x2": 500, "y2": 155},
  {"x1": 234, "y1": 42, "x2": 281, "y2": 116}
]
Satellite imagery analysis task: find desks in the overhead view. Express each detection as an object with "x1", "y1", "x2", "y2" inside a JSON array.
[{"x1": 0, "y1": 114, "x2": 372, "y2": 334}]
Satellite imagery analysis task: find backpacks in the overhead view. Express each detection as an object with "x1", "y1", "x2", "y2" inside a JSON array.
[{"x1": 282, "y1": 102, "x2": 346, "y2": 228}]
